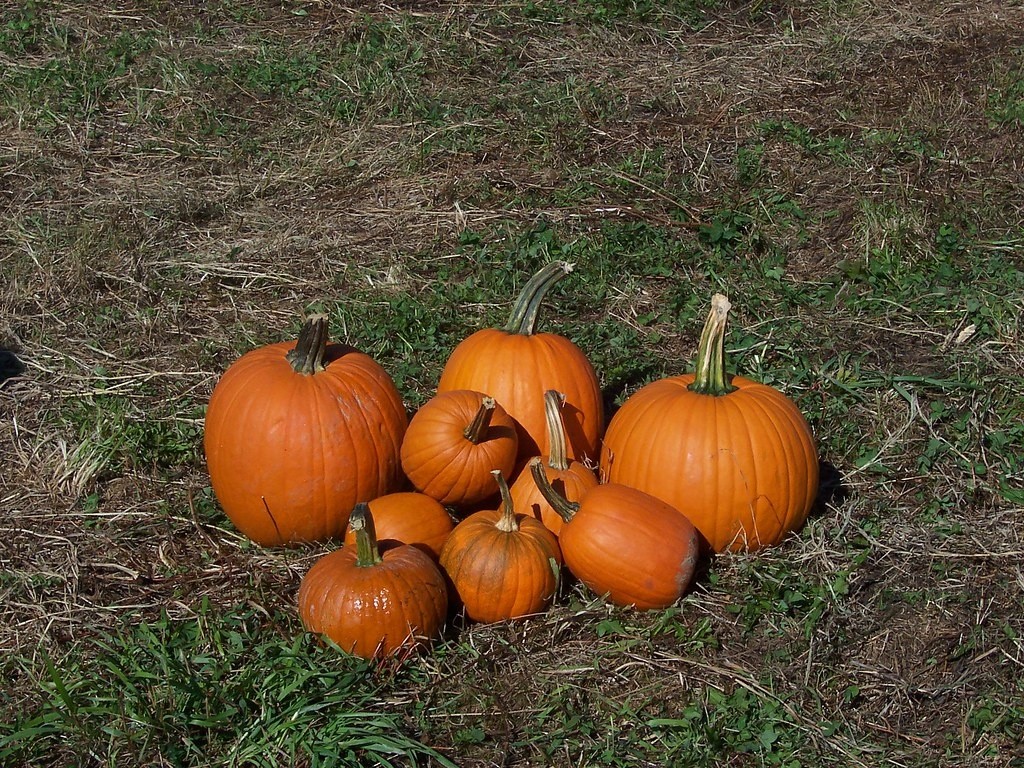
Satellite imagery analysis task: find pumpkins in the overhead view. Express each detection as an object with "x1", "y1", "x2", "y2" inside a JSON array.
[{"x1": 203, "y1": 261, "x2": 819, "y2": 659}]
